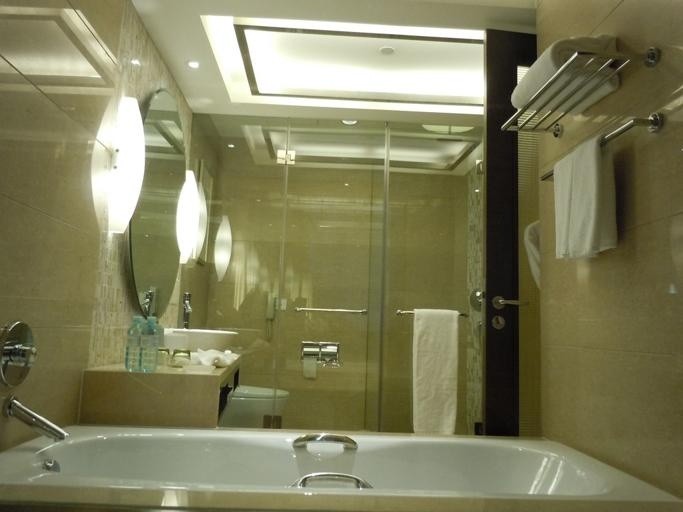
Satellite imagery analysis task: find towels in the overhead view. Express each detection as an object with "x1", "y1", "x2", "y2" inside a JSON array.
[
  {"x1": 411, "y1": 307, "x2": 460, "y2": 437},
  {"x1": 510, "y1": 32, "x2": 622, "y2": 117},
  {"x1": 552, "y1": 130, "x2": 619, "y2": 260}
]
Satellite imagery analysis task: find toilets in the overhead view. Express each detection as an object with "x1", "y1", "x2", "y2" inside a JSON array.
[{"x1": 219, "y1": 383, "x2": 290, "y2": 427}]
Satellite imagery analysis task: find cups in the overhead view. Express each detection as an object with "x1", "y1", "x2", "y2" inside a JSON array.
[{"x1": 156, "y1": 348, "x2": 191, "y2": 368}]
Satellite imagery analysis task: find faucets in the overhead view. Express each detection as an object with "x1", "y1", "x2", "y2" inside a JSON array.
[
  {"x1": 8, "y1": 395, "x2": 69, "y2": 441},
  {"x1": 183, "y1": 293, "x2": 192, "y2": 327},
  {"x1": 141, "y1": 289, "x2": 158, "y2": 318}
]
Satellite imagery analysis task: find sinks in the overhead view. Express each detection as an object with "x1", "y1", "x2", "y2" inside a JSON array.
[
  {"x1": 162, "y1": 328, "x2": 240, "y2": 352},
  {"x1": 221, "y1": 327, "x2": 267, "y2": 353}
]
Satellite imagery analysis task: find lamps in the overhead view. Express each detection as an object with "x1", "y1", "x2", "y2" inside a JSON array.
[
  {"x1": 175, "y1": 167, "x2": 205, "y2": 266},
  {"x1": 213, "y1": 215, "x2": 233, "y2": 282},
  {"x1": 107, "y1": 93, "x2": 147, "y2": 234}
]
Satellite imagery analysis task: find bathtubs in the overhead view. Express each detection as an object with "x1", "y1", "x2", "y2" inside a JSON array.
[{"x1": 0, "y1": 424, "x2": 683, "y2": 511}]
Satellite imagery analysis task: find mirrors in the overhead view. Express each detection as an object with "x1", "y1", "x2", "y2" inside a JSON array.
[{"x1": 128, "y1": 86, "x2": 186, "y2": 321}]
